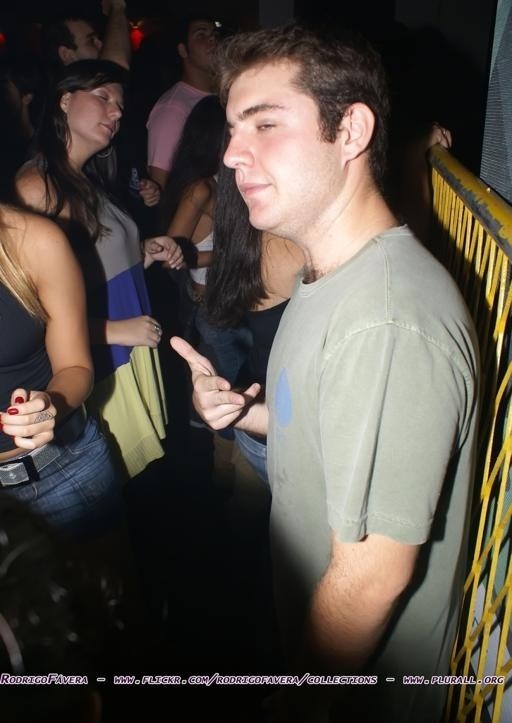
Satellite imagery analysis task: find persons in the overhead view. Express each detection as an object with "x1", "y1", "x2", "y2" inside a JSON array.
[
  {"x1": 0, "y1": 1, "x2": 456, "y2": 633},
  {"x1": 170, "y1": 25, "x2": 481, "y2": 721}
]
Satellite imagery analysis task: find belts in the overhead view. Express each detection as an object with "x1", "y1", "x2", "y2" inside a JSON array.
[{"x1": 0, "y1": 444, "x2": 62, "y2": 489}]
